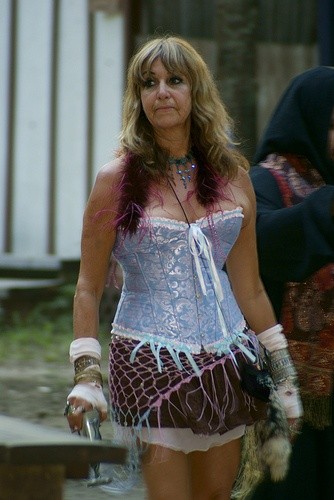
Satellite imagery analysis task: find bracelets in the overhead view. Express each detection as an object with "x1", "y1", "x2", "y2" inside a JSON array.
[
  {"x1": 256, "y1": 323, "x2": 298, "y2": 382},
  {"x1": 68, "y1": 337, "x2": 101, "y2": 384}
]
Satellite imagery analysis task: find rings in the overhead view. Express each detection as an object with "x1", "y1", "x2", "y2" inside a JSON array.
[{"x1": 63, "y1": 405, "x2": 85, "y2": 414}]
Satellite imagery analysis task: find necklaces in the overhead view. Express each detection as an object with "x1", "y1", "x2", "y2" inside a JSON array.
[{"x1": 155, "y1": 150, "x2": 195, "y2": 189}]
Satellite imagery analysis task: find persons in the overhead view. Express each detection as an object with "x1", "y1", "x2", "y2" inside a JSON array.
[
  {"x1": 221, "y1": 64, "x2": 334, "y2": 500},
  {"x1": 63, "y1": 37, "x2": 304, "y2": 500}
]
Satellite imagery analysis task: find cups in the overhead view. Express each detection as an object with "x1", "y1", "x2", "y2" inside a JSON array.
[{"x1": 72, "y1": 409, "x2": 114, "y2": 489}]
